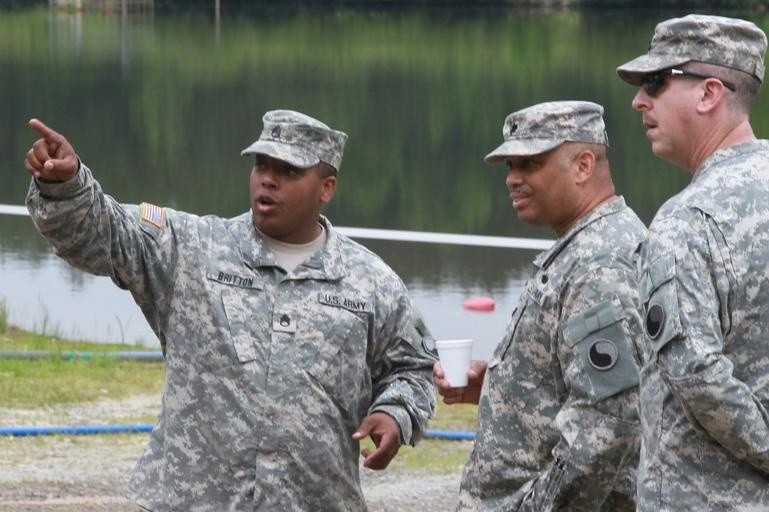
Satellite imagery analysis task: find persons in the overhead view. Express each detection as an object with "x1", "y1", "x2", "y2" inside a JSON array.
[
  {"x1": 430, "y1": 101, "x2": 647, "y2": 512},
  {"x1": 616, "y1": 13, "x2": 769, "y2": 511},
  {"x1": 19, "y1": 109, "x2": 440, "y2": 511}
]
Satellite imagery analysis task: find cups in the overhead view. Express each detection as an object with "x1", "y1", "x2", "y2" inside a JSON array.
[{"x1": 435, "y1": 338, "x2": 474, "y2": 388}]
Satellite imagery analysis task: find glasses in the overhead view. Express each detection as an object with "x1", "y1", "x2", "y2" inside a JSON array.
[{"x1": 642, "y1": 69, "x2": 736, "y2": 96}]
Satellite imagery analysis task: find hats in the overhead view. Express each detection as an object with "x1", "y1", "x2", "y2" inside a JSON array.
[
  {"x1": 616, "y1": 13, "x2": 767, "y2": 85},
  {"x1": 241, "y1": 109, "x2": 349, "y2": 171},
  {"x1": 482, "y1": 100, "x2": 610, "y2": 165}
]
